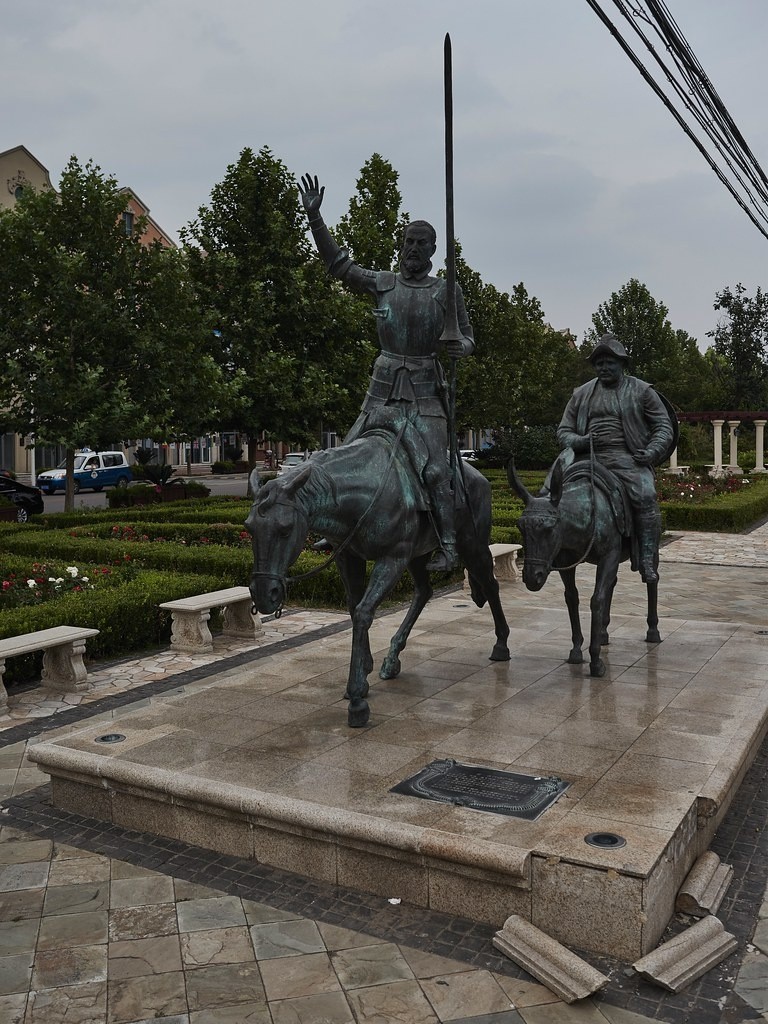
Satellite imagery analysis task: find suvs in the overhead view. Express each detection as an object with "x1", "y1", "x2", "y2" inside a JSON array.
[{"x1": 38, "y1": 448, "x2": 133, "y2": 496}]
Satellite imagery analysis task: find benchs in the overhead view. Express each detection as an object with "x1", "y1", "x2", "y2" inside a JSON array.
[
  {"x1": 156, "y1": 584, "x2": 267, "y2": 655},
  {"x1": 703, "y1": 463, "x2": 730, "y2": 475},
  {"x1": 677, "y1": 465, "x2": 691, "y2": 475},
  {"x1": 0, "y1": 625, "x2": 101, "y2": 716},
  {"x1": 462, "y1": 544, "x2": 522, "y2": 591}
]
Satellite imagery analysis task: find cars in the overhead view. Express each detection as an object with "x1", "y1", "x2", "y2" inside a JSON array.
[
  {"x1": 457, "y1": 448, "x2": 484, "y2": 462},
  {"x1": 276, "y1": 452, "x2": 311, "y2": 477},
  {"x1": 0, "y1": 473, "x2": 45, "y2": 525}
]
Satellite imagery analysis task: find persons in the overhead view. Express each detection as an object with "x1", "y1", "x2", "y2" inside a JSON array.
[
  {"x1": 297, "y1": 171, "x2": 477, "y2": 568},
  {"x1": 85, "y1": 459, "x2": 98, "y2": 469},
  {"x1": 540, "y1": 334, "x2": 678, "y2": 586}
]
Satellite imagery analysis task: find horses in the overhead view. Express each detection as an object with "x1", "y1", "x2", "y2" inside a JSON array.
[
  {"x1": 502, "y1": 458, "x2": 663, "y2": 680},
  {"x1": 244, "y1": 437, "x2": 513, "y2": 727}
]
